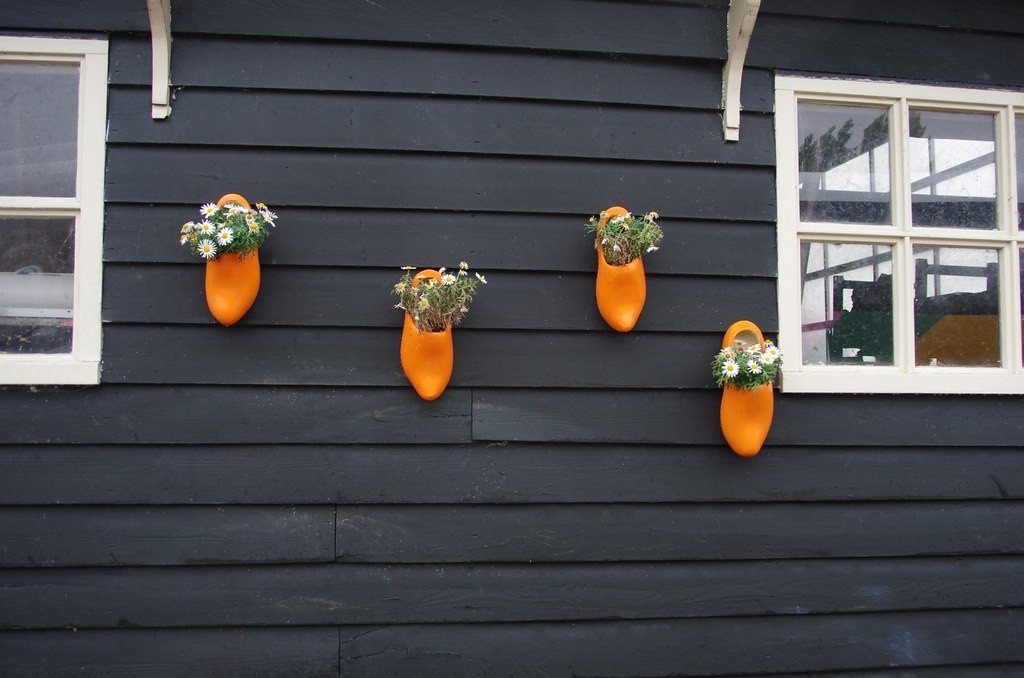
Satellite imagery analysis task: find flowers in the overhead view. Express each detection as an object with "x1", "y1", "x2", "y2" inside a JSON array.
[
  {"x1": 584, "y1": 209, "x2": 664, "y2": 267},
  {"x1": 177, "y1": 195, "x2": 278, "y2": 261},
  {"x1": 710, "y1": 339, "x2": 784, "y2": 394},
  {"x1": 388, "y1": 260, "x2": 488, "y2": 337}
]
